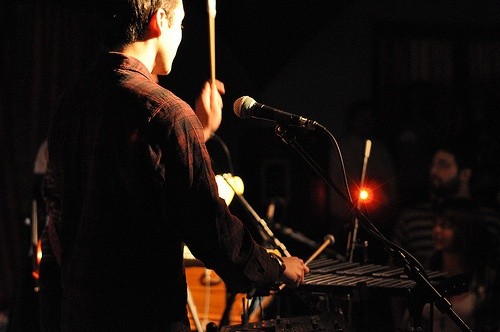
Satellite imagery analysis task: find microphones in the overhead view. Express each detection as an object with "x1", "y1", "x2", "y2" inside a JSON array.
[{"x1": 232, "y1": 95, "x2": 319, "y2": 131}]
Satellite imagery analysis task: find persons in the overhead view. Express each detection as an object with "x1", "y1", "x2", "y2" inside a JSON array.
[
  {"x1": 389, "y1": 141, "x2": 499, "y2": 331},
  {"x1": 420, "y1": 241, "x2": 483, "y2": 331},
  {"x1": 35, "y1": 0, "x2": 314, "y2": 332}
]
